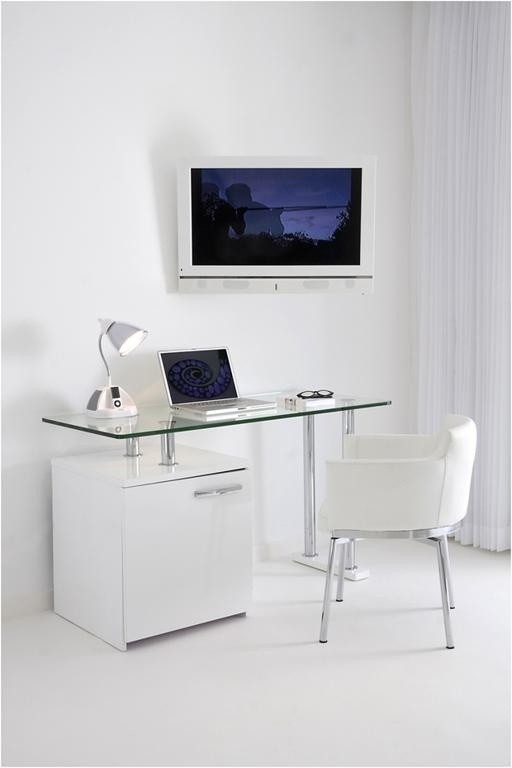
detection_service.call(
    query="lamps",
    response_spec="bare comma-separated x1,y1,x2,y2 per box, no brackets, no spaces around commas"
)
81,315,154,420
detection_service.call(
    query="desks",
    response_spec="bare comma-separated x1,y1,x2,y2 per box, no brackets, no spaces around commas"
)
38,381,394,658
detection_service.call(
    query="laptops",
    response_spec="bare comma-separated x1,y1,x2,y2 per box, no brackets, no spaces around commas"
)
157,346,277,415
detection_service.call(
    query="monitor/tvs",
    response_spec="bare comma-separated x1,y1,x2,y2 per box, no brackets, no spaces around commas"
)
178,155,375,278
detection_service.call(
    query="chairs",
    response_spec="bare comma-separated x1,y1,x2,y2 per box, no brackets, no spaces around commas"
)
313,411,482,653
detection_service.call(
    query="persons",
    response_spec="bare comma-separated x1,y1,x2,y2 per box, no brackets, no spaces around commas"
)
200,180,248,256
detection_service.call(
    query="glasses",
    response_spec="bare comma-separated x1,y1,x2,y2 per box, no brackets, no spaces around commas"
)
296,389,336,399
